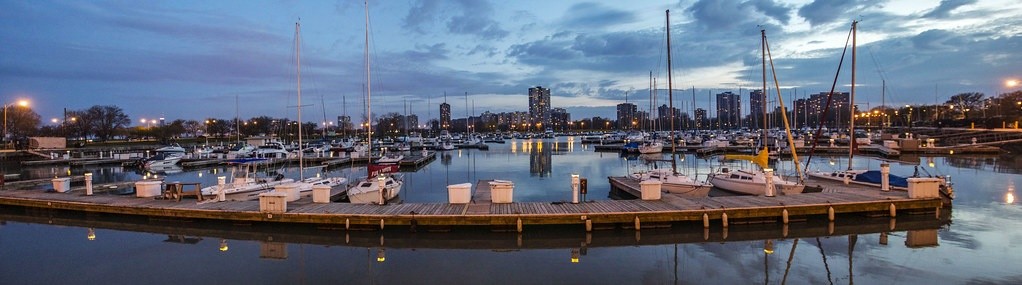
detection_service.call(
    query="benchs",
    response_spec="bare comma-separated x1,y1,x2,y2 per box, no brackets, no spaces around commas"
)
162,233,204,247
163,181,203,203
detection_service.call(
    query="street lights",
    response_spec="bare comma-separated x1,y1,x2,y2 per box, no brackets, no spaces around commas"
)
141,118,156,140
4,101,27,149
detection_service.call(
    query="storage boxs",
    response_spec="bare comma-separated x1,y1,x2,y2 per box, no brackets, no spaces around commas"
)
258,193,288,213
311,185,331,203
639,181,663,201
135,181,162,198
258,242,289,262
906,178,942,198
488,180,515,204
51,178,71,193
447,183,472,204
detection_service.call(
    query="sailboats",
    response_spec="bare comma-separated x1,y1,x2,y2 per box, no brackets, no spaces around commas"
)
803,19,955,208
707,28,807,197
627,7,714,197
345,1,403,205
268,18,349,204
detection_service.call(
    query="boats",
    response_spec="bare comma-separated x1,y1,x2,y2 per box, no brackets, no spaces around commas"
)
143,89,484,174
199,94,295,202
581,69,939,154
